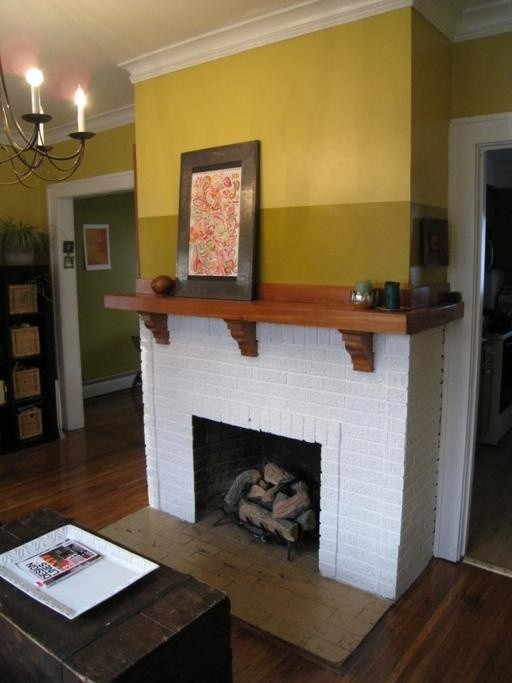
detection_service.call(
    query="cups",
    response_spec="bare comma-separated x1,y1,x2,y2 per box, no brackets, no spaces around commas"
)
384,281,400,309
374,288,384,308
356,280,371,293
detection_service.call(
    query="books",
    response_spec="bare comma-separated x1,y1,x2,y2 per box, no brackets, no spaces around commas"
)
16,538,103,589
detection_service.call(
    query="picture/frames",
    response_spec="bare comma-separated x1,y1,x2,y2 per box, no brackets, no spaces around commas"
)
174,139,260,301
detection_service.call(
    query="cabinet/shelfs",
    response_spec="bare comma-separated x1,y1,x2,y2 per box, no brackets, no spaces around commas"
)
0,265,58,456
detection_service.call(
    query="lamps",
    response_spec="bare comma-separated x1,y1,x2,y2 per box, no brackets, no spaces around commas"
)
0,55,95,187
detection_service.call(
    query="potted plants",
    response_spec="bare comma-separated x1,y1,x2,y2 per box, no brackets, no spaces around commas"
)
0,218,42,264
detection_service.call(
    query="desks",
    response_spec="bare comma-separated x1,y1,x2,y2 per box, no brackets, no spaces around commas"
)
0,507,231,681
